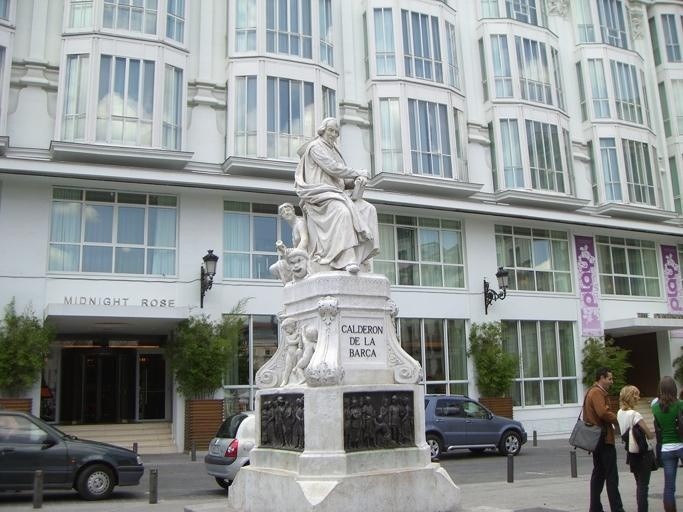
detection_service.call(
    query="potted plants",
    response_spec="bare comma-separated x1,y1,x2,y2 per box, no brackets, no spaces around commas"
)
579,335,632,412
161,295,255,452
466,321,520,421
0,294,52,414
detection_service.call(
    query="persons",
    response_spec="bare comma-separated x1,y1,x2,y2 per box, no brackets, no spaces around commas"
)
581,365,627,512
260,393,413,449
646,375,683,512
650,396,664,467
268,202,306,285
277,318,301,386
615,383,656,512
290,323,318,384
297,117,376,272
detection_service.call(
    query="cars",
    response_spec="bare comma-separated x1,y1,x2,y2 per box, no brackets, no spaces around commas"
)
424,393,528,462
204,408,258,494
0,408,145,501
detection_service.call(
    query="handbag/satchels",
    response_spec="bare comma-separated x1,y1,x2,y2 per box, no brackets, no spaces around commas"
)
628,410,640,454
646,440,659,472
569,419,602,452
675,409,683,441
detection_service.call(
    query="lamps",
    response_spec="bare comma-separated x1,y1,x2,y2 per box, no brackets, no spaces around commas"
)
199,248,218,310
481,266,509,314
